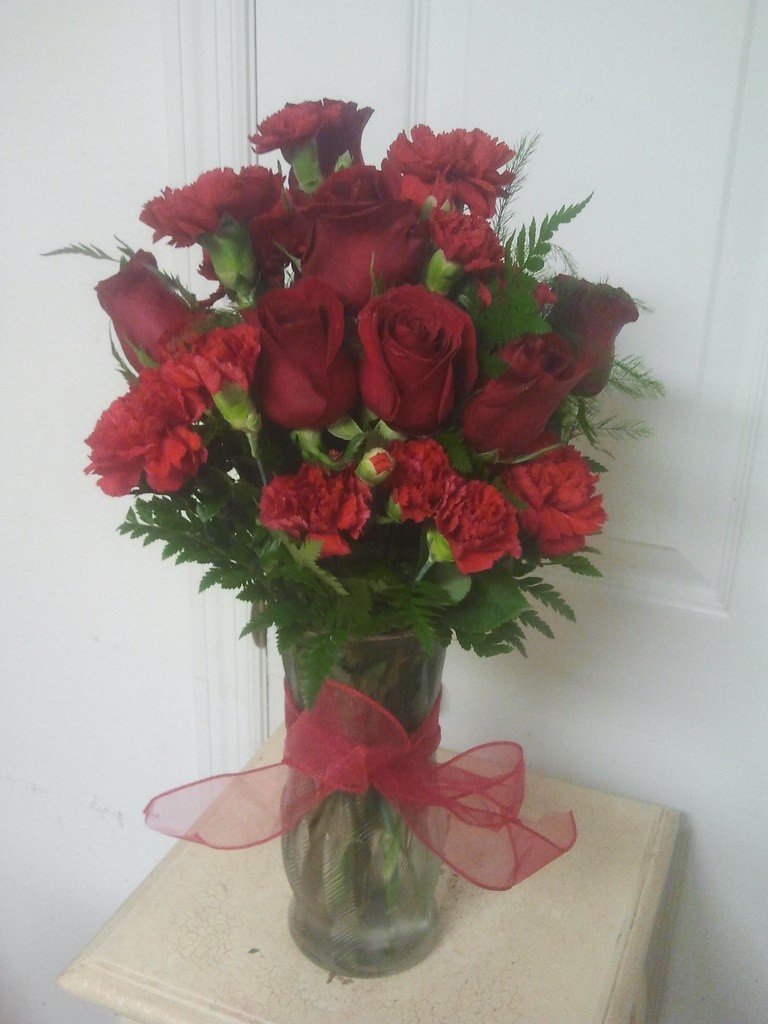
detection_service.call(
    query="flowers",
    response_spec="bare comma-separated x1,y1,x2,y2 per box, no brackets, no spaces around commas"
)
55,106,676,920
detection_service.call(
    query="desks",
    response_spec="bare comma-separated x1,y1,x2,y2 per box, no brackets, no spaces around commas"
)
57,744,687,1024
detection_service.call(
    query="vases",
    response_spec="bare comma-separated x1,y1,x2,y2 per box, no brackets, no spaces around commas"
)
274,620,443,977
